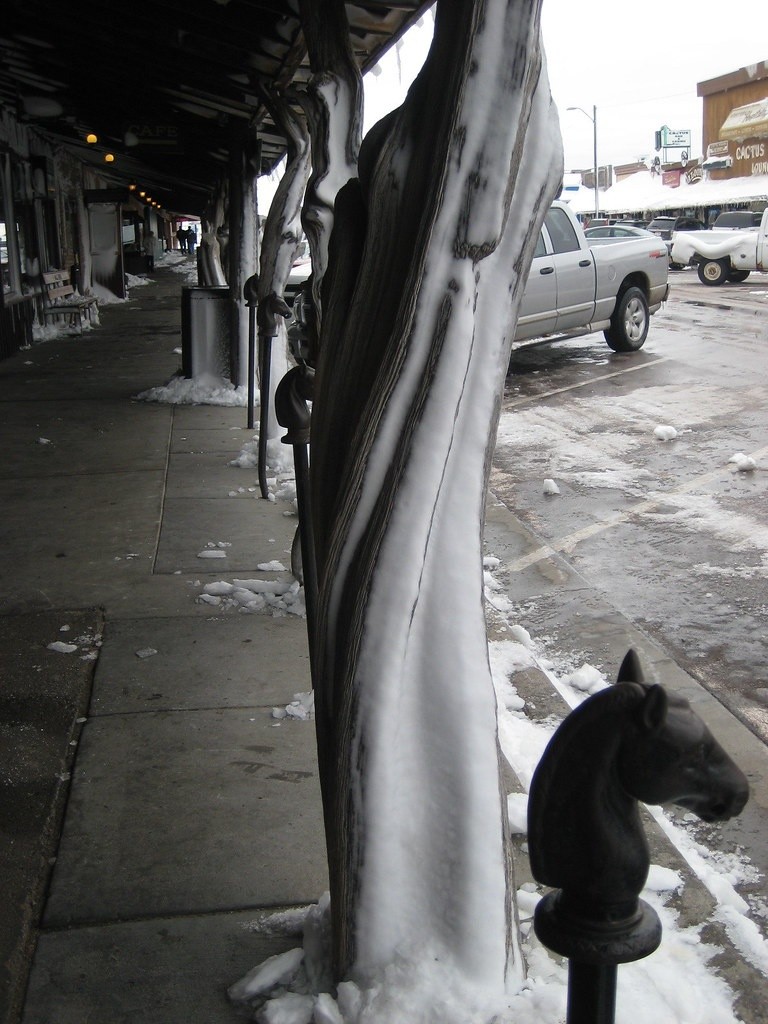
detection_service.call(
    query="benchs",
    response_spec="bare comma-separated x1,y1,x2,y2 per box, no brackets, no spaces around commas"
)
40,269,100,332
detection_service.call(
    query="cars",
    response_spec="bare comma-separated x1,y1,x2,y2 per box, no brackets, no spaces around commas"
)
284,232,312,307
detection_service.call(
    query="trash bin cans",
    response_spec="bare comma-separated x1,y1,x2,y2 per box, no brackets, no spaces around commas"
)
179,284,234,383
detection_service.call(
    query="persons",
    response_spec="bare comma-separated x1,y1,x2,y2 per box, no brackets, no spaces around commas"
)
176,226,187,255
185,226,195,254
142,231,155,268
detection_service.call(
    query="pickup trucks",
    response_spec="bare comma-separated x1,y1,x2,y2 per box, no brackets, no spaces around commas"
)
669,206,768,287
287,199,670,366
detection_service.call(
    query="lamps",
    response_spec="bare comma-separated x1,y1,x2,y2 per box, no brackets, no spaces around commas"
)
146,192,152,202
101,154,115,165
85,133,99,148
139,186,147,197
126,177,138,190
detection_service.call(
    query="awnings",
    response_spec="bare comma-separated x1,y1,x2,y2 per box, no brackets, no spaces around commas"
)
718,98,768,139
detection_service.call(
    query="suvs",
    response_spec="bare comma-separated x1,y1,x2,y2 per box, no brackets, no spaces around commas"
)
567,216,709,270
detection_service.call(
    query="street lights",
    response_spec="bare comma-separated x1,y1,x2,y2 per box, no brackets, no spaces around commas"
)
566,105,599,219
